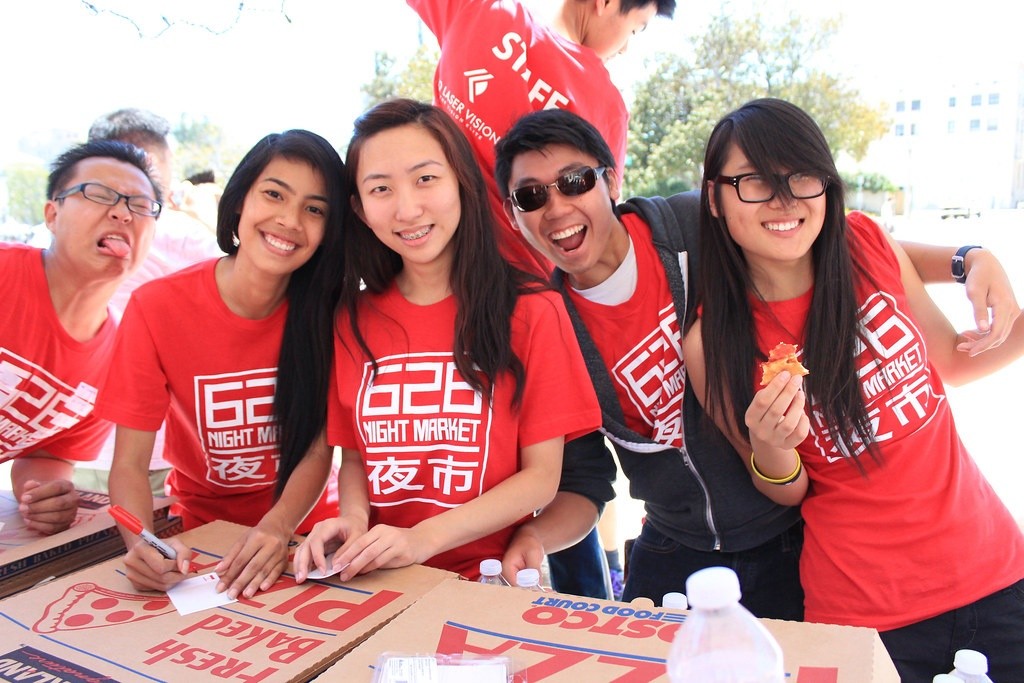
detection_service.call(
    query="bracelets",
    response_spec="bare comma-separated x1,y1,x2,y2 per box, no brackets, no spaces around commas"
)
751,451,802,485
951,246,982,283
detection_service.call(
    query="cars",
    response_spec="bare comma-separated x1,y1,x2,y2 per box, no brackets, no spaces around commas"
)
940,199,982,220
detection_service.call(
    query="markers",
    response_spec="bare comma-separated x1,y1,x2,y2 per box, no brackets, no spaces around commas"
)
107,505,197,575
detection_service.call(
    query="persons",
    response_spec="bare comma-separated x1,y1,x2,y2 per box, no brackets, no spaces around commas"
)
677,99,1024,683
409,0,675,599
25,105,228,501
91,128,349,598
495,111,1023,620
0,137,164,535
291,98,604,582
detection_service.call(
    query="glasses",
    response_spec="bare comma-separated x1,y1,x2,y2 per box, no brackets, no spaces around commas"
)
712,168,831,203
52,182,163,220
507,165,607,212
